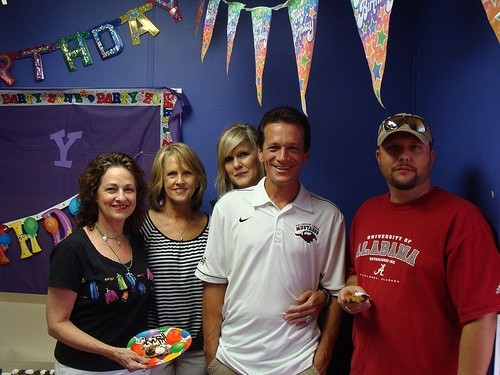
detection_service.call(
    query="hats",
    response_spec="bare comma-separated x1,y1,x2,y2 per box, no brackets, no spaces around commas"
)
377,112,432,146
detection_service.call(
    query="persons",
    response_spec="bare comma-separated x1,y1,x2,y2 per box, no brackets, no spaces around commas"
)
195,106,345,375
46,153,155,375
135,143,211,375
337,113,500,375
210,121,332,326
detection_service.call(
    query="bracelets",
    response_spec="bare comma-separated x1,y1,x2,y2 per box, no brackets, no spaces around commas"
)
319,289,330,307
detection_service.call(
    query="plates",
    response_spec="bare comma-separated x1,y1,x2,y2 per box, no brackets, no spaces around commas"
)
126,326,192,368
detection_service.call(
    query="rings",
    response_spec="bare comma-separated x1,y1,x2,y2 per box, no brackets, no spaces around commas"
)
307,315,312,324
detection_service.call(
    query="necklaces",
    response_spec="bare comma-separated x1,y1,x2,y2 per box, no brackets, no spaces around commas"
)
94,223,133,276
164,211,190,241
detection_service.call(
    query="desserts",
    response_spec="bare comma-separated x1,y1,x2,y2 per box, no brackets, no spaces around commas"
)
142,333,168,358
344,291,372,308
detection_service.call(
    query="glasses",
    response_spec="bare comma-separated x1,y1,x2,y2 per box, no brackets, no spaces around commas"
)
378,115,428,133
